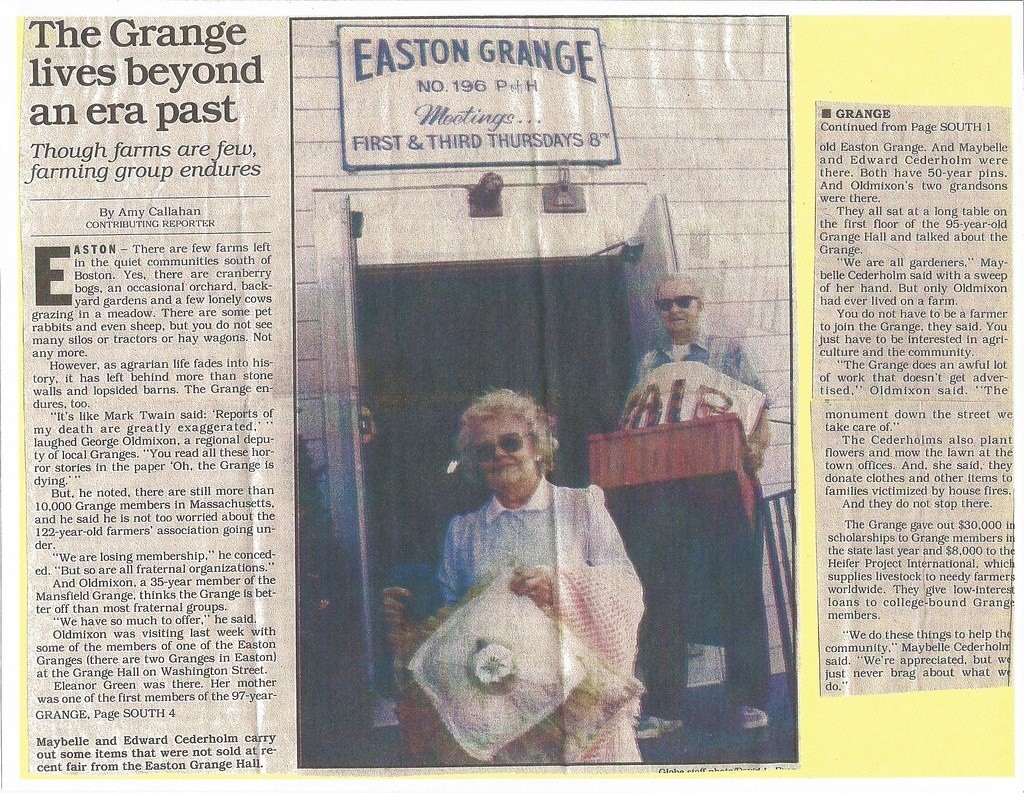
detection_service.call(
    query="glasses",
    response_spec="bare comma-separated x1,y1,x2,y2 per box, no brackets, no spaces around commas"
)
471,431,530,465
653,294,699,312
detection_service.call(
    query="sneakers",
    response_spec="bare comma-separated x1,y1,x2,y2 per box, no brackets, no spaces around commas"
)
635,715,685,740
740,705,769,730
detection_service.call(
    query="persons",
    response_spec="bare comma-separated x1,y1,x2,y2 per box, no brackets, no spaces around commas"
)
635,273,770,741
381,388,646,764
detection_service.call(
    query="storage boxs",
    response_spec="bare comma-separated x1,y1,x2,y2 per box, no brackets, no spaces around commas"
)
587,412,766,549
